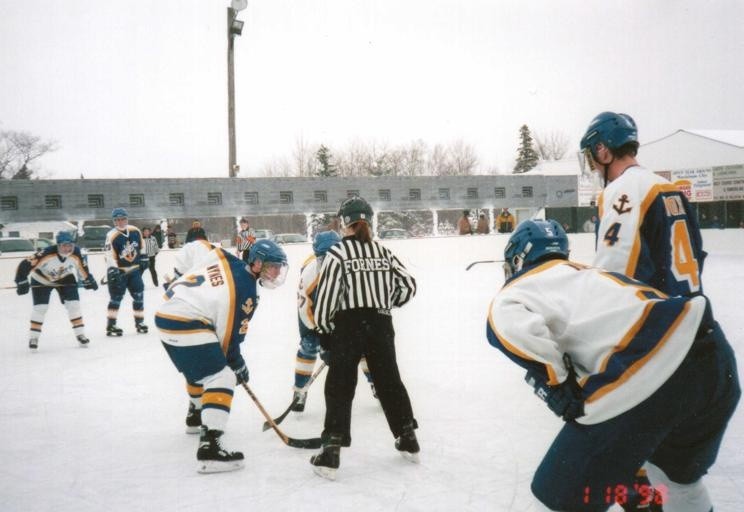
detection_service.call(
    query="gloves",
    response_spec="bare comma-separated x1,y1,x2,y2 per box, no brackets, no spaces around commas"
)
140,257,152,273
15,279,29,295
84,275,98,291
235,365,248,387
106,266,119,281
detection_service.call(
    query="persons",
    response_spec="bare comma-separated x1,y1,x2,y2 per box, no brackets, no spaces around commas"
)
309,196,422,481
495,208,516,233
477,214,489,234
168,226,176,248
235,218,257,261
291,228,384,412
583,215,597,233
103,207,149,336
561,221,573,233
14,228,98,353
139,226,160,288
151,225,163,249
154,238,289,474
458,210,473,234
185,219,207,243
575,110,710,298
486,218,743,511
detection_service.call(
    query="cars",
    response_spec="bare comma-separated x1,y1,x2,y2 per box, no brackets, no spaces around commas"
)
255,229,274,243
27,237,54,249
380,228,409,238
80,225,112,253
274,233,308,244
0,237,38,261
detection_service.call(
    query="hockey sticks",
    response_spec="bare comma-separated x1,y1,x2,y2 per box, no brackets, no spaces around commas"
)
101,267,144,283
262,363,327,431
240,378,321,449
466,258,504,271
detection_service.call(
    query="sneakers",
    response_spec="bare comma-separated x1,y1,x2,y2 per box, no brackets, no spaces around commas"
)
106,320,122,332
197,430,244,462
291,390,306,412
29,338,38,349
394,431,419,454
186,410,203,429
76,333,90,344
309,444,341,470
135,321,148,330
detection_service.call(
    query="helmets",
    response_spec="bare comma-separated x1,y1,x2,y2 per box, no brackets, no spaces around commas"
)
502,220,570,278
337,197,374,230
246,240,288,289
313,231,341,256
56,231,76,256
113,209,127,229
575,111,639,178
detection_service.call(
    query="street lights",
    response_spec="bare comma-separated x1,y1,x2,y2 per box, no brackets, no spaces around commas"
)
227,0,246,178
